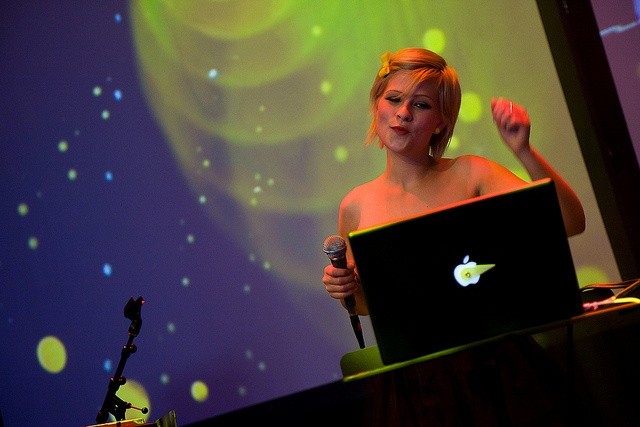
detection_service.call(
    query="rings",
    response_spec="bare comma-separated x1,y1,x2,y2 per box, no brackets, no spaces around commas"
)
509,101,513,112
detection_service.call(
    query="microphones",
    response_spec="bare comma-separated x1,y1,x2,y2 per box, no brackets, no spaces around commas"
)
323,234,365,349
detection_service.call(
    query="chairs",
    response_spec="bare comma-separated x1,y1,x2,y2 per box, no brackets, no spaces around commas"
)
340,344,385,377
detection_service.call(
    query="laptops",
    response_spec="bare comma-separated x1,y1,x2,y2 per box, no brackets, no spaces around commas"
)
348,176,586,366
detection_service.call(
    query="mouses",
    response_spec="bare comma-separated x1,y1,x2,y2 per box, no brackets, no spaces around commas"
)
582,288,615,310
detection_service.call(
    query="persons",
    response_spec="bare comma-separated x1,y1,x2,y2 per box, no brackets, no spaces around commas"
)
322,47,586,317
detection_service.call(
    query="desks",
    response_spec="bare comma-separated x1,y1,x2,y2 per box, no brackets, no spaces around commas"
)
178,295,640,427
